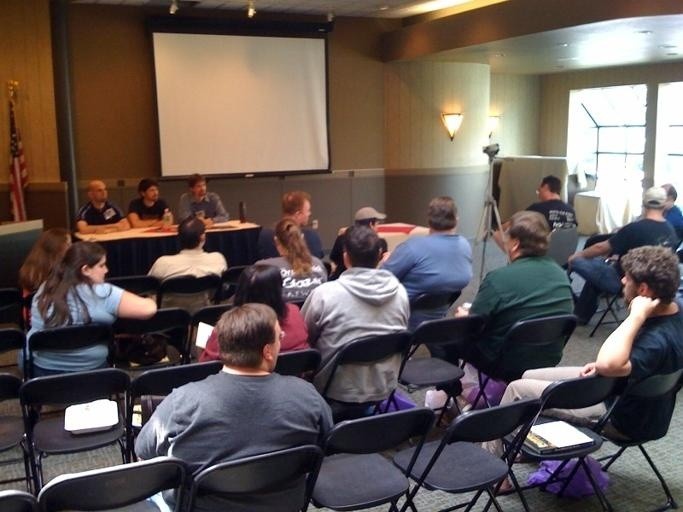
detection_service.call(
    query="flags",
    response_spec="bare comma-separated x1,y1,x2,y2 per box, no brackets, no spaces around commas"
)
8,98,29,222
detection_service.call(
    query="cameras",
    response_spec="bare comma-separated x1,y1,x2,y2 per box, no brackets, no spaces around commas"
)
484,142,499,155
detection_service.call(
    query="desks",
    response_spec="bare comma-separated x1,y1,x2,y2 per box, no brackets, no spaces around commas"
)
74,220,261,277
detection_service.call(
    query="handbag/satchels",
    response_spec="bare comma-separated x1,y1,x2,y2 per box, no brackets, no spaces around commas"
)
112,329,169,365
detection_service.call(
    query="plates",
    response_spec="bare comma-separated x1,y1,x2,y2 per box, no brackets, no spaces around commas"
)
64,399,119,435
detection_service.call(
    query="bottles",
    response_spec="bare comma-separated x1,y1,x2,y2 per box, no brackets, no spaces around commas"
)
162,208,173,229
239,201,247,223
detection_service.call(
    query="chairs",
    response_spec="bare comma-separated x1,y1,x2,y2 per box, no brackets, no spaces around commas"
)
1,264,322,512
409,289,462,312
311,314,681,511
547,223,681,338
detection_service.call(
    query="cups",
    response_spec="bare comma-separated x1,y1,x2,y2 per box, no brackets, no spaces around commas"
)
462,303,472,311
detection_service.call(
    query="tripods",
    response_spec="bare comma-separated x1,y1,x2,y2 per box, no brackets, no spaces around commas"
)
468,159,513,285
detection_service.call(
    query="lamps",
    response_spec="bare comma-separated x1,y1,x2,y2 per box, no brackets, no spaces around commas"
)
441,112,463,141
487,116,500,138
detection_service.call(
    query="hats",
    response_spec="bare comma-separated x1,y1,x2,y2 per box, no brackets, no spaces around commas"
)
644,187,670,208
354,206,388,221
